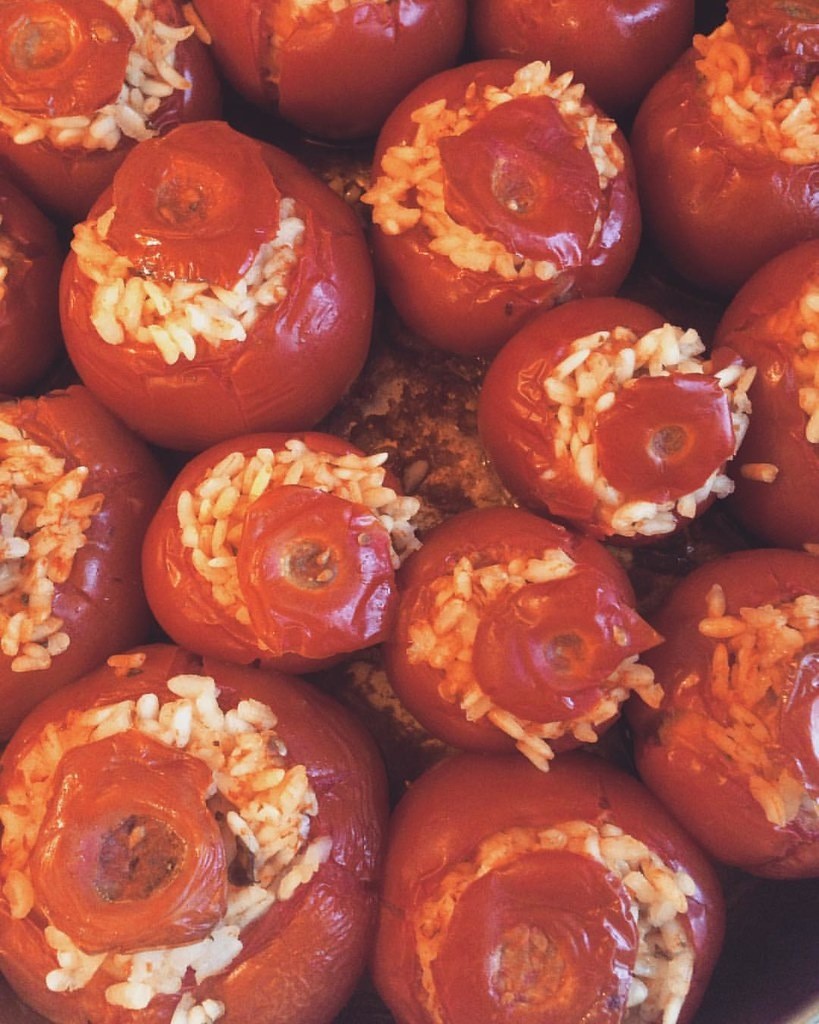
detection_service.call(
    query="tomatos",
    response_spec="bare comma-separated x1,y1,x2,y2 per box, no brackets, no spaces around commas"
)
0,0,819,1024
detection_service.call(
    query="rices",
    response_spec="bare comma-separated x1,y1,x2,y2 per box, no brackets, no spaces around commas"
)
1,0,819,1024
